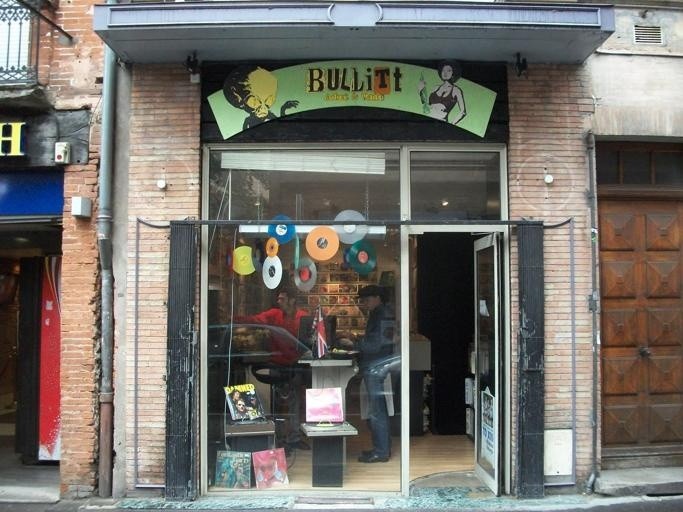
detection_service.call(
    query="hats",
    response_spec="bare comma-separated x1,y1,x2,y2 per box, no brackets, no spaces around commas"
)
342,344,400,435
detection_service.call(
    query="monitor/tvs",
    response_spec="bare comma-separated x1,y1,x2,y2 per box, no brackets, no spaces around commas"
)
214,449,251,490
304,386,343,424
252,447,289,490
298,422,358,436
221,382,265,421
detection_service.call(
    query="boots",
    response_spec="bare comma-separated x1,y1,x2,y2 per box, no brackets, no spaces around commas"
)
206,321,315,412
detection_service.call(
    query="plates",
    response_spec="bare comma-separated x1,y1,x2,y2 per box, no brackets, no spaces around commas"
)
300,422,358,488
226,419,275,487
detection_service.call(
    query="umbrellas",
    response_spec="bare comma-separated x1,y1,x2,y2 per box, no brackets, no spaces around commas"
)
283,439,311,450
358,452,388,463
362,448,391,457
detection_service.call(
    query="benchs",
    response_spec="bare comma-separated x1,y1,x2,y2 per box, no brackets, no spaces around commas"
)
311,303,327,358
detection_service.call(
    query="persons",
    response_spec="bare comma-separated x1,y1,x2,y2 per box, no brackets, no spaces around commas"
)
353,284,401,465
228,388,260,420
228,289,318,452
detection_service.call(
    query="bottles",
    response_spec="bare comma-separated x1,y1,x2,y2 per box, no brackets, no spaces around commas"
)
356,285,382,299
